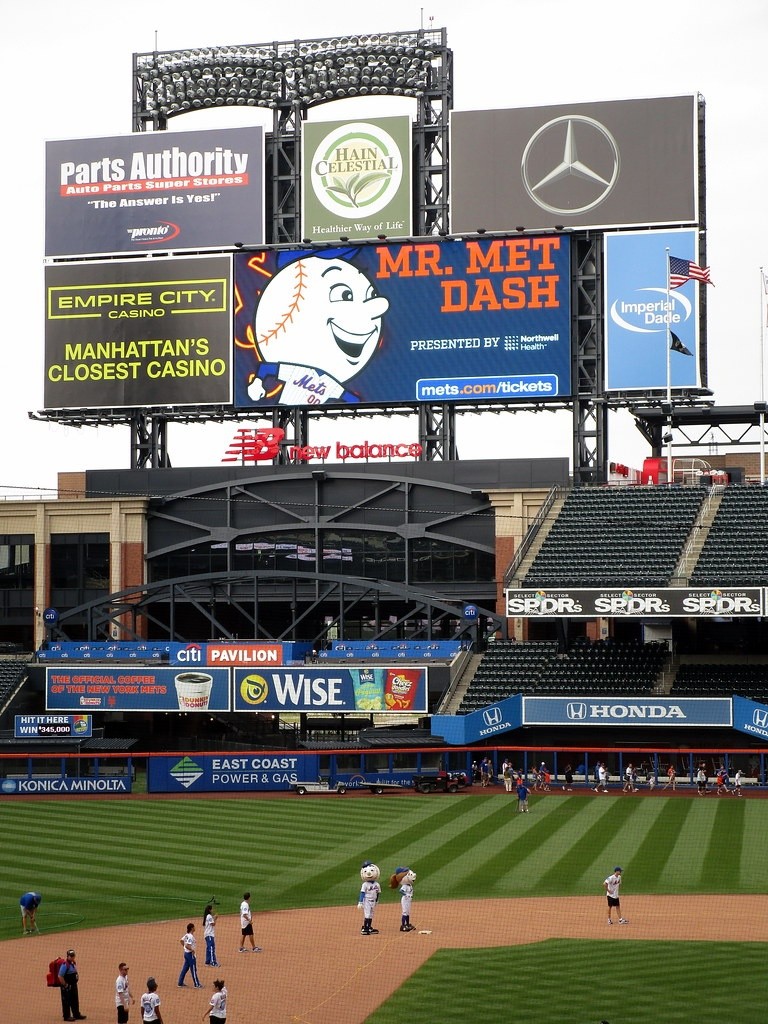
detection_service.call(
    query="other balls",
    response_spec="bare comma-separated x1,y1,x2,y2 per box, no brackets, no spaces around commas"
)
30,930,32,933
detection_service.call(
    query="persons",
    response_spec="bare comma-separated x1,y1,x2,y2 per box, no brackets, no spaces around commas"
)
715,764,731,796
237,893,263,953
591,760,610,792
176,922,203,988
622,763,638,793
202,905,221,967
731,770,745,797
356,861,381,934
661,764,677,791
389,866,417,931
649,775,655,791
601,867,629,924
696,761,712,797
114,962,136,1024
202,978,228,1024
471,757,551,793
561,765,573,791
58,949,86,1022
19,892,41,935
516,783,532,813
140,976,164,1024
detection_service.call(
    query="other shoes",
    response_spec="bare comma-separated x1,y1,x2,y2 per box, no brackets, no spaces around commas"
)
73,1013,86,1020
471,780,744,813
30,930,34,933
360,926,369,935
406,924,416,930
608,920,612,925
619,919,629,924
23,931,29,935
64,1016,76,1021
368,927,379,934
400,925,410,932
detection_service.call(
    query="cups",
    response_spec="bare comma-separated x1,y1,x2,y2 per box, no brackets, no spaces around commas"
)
174,672,213,710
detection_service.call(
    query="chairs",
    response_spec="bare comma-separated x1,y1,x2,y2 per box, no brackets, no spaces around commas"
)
456,483,768,716
0,659,30,704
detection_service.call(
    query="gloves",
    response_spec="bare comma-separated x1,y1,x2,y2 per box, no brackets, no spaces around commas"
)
358,902,363,909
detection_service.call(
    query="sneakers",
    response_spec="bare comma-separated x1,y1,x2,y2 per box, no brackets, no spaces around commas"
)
204,962,212,967
177,983,188,988
252,947,262,953
239,947,249,952
212,964,221,968
194,984,205,989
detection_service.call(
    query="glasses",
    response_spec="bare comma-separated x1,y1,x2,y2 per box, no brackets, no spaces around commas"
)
123,968,129,971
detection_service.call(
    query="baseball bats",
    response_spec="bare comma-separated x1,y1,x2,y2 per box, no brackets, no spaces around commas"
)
34,921,43,935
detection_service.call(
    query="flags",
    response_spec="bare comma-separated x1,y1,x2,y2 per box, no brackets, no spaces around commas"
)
669,256,715,289
669,331,694,356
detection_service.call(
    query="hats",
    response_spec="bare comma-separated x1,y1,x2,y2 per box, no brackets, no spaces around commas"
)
541,762,545,765
615,867,623,872
35,895,41,904
67,949,75,957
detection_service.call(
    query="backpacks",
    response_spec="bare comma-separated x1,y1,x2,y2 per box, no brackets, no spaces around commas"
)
46,957,70,987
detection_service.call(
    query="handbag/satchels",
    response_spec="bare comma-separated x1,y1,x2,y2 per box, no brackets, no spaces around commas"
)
624,775,630,781
